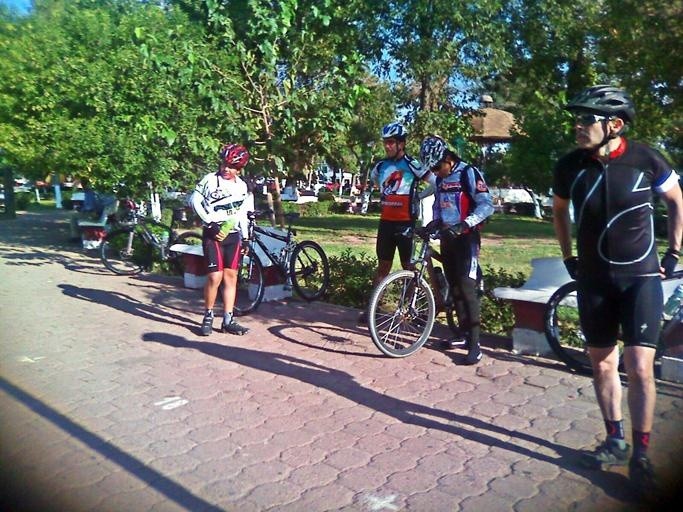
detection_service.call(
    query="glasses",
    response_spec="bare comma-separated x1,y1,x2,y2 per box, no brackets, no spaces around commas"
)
430,155,446,171
382,140,396,145
222,161,236,169
572,110,618,125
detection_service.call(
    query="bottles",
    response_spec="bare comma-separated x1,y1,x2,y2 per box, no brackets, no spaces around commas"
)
663,283,683,317
434,266,447,301
216,217,235,241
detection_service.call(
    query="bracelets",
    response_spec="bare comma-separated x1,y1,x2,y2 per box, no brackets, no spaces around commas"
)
667,248,683,257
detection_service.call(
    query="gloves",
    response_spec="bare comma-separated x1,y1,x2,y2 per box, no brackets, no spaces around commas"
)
445,223,464,240
426,220,440,231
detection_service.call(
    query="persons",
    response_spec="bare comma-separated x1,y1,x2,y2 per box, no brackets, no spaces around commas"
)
71,177,97,239
189,144,250,335
552,84,683,501
360,123,438,323
420,134,495,365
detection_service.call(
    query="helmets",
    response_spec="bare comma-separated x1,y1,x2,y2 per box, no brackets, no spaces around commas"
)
566,84,635,123
382,122,407,139
221,144,248,168
420,135,446,167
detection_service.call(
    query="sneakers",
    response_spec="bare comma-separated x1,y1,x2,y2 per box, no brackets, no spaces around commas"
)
629,457,653,494
221,323,250,335
578,441,629,465
465,351,482,364
201,318,212,335
360,310,380,322
449,336,480,348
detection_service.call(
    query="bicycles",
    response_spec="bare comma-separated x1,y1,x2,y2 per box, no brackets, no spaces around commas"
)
99,198,203,278
218,209,329,316
543,251,682,376
366,225,484,359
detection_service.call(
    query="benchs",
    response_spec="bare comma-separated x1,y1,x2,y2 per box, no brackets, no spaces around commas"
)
169,226,292,303
76,208,110,249
494,257,683,386
70,193,85,209
